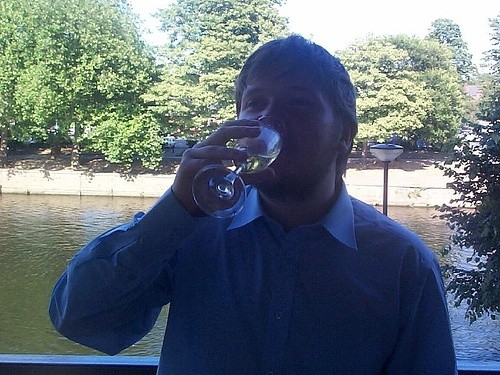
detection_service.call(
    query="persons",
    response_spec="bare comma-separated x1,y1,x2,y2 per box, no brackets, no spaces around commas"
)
51,36,458,375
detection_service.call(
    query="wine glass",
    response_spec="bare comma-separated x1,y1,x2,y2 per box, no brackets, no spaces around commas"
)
192,122,282,218
